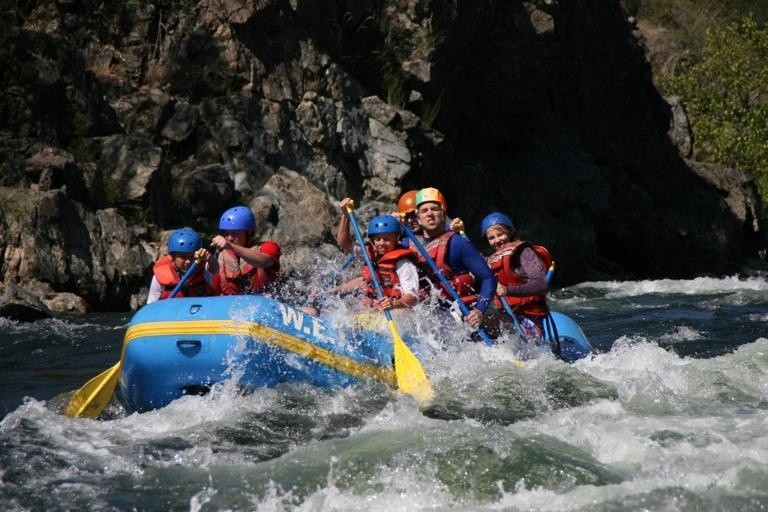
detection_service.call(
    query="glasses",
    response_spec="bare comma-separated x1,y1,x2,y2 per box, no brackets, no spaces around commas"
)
404,213,416,219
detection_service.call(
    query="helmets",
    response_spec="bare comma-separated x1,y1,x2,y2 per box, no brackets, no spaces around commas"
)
415,187,447,211
398,190,418,216
368,215,402,238
168,228,202,254
481,212,513,233
219,206,256,241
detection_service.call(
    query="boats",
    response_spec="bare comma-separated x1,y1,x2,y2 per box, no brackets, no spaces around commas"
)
117,293,593,415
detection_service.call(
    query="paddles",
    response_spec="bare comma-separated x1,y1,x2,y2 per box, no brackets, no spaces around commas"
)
339,200,431,401
64,254,204,419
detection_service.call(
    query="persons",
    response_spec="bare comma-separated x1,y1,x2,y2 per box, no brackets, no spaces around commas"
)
449,212,547,344
398,187,496,328
337,197,419,311
209,206,281,296
146,227,219,305
396,190,421,233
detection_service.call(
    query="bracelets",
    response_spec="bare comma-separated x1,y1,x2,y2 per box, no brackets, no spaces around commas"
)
353,245,358,257
392,297,395,308
